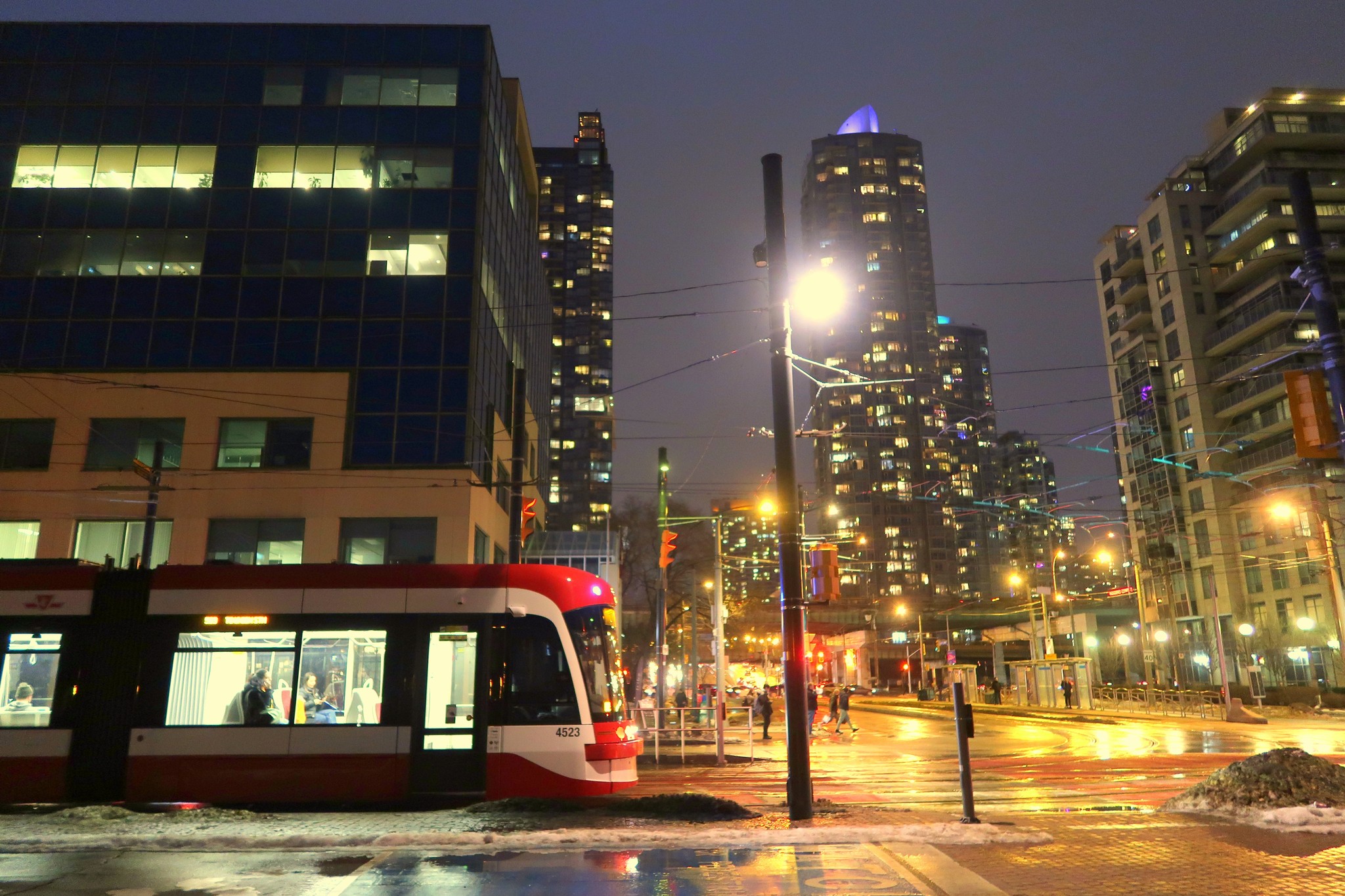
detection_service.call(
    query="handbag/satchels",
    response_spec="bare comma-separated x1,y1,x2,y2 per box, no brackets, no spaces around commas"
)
823,715,832,724
272,679,292,720
754,694,764,714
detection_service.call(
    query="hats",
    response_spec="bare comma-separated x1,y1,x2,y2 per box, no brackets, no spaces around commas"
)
15,683,33,698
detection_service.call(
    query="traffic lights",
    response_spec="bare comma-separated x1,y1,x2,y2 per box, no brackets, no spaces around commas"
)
846,650,855,669
818,651,824,663
924,664,930,671
520,495,536,549
816,664,823,670
903,663,908,670
1221,686,1225,697
659,529,678,568
1256,655,1266,664
806,651,812,663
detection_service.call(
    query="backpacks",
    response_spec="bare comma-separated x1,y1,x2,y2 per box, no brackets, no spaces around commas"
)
742,698,753,711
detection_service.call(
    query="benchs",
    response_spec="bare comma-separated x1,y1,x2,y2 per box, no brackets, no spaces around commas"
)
0,707,51,727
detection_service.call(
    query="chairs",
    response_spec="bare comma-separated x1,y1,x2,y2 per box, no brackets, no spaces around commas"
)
344,678,382,724
272,679,293,724
222,693,245,724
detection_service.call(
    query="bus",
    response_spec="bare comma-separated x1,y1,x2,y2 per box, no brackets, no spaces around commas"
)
0,557,645,801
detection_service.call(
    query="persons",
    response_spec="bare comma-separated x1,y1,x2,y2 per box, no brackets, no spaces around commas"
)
1061,677,1072,709
818,689,840,732
298,671,337,723
652,686,657,699
757,684,773,739
241,669,282,725
675,688,688,719
835,688,859,735
745,689,756,735
903,684,909,695
4,682,37,711
803,682,818,734
991,677,1001,705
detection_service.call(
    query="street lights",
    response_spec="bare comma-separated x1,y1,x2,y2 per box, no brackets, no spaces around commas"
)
743,634,781,666
1085,637,1102,685
1296,617,1318,689
896,604,912,693
1097,532,1233,718
657,446,673,741
753,152,848,819
715,496,783,767
1052,550,1079,659
1154,631,1167,690
1117,634,1132,689
1238,623,1253,667
1107,532,1132,601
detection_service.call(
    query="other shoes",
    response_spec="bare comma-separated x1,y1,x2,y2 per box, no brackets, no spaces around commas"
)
853,728,859,733
835,730,843,734
809,734,816,738
763,735,772,739
1070,707,1073,709
